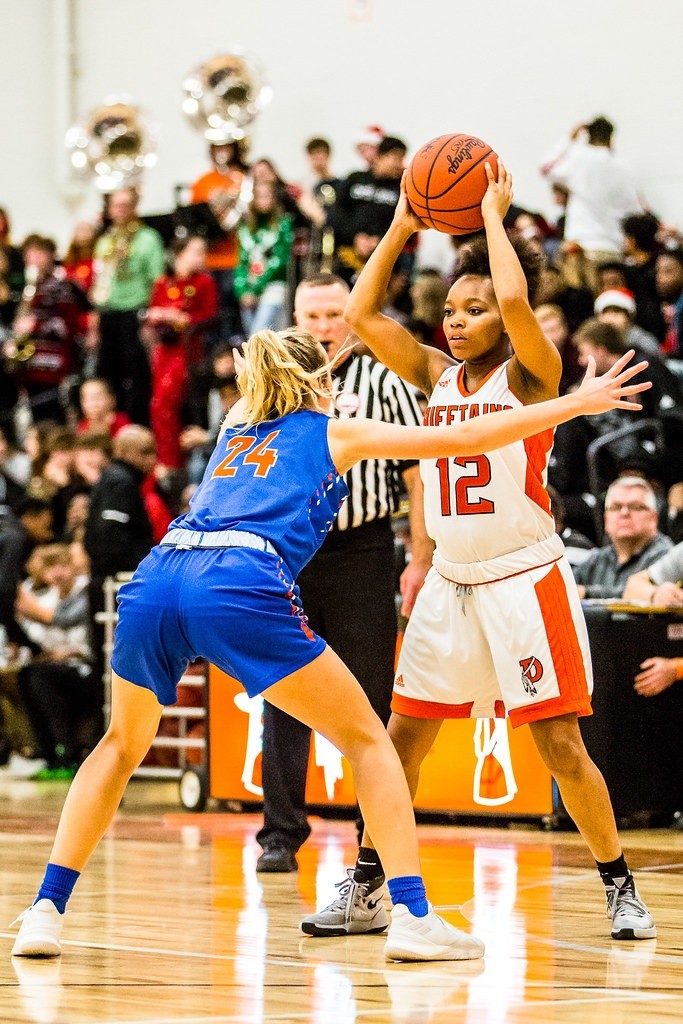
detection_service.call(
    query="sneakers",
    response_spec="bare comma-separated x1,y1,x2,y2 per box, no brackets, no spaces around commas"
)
384,901,485,960
10,899,66,956
301,869,393,937
600,869,657,939
256,838,298,872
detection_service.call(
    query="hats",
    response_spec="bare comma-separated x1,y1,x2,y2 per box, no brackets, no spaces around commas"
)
357,127,385,147
624,215,659,252
593,286,636,314
567,243,584,256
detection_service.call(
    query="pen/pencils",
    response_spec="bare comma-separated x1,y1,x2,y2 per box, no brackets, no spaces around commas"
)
675,580,683,588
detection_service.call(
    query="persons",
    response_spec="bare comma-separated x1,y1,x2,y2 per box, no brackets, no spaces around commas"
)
1,115,683,871
293,163,653,940
14,328,656,960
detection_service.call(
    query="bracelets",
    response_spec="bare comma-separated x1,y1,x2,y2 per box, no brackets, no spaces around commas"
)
649,591,656,604
674,656,683,680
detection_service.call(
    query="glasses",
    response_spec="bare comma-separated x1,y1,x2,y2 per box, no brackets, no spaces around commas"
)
604,501,650,515
140,446,156,455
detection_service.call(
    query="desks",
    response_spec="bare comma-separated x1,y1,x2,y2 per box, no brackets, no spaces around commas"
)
557,598,683,831
205,602,565,830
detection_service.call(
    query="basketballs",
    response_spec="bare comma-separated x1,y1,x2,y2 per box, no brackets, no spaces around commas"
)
406,132,500,236
